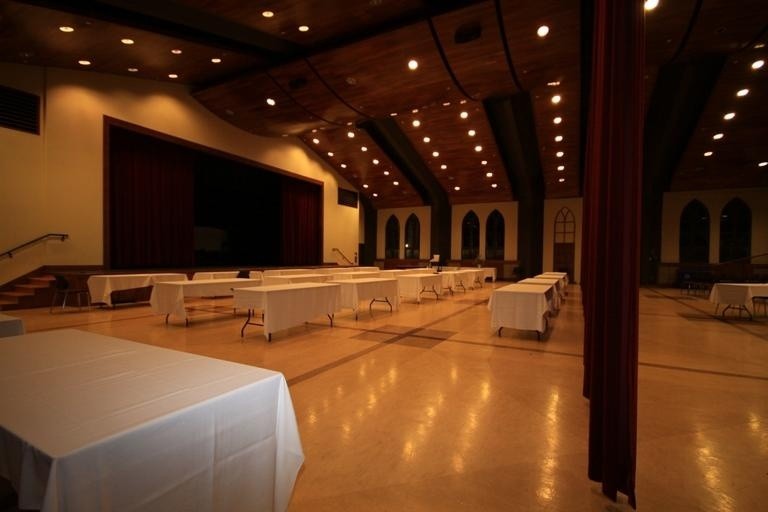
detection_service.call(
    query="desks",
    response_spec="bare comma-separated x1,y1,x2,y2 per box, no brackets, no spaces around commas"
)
493,271,569,341
0,329,307,512
0,313,23,337
710,282,768,323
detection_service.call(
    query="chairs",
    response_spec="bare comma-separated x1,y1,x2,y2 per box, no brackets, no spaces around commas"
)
429,254,441,268
50,275,91,313
